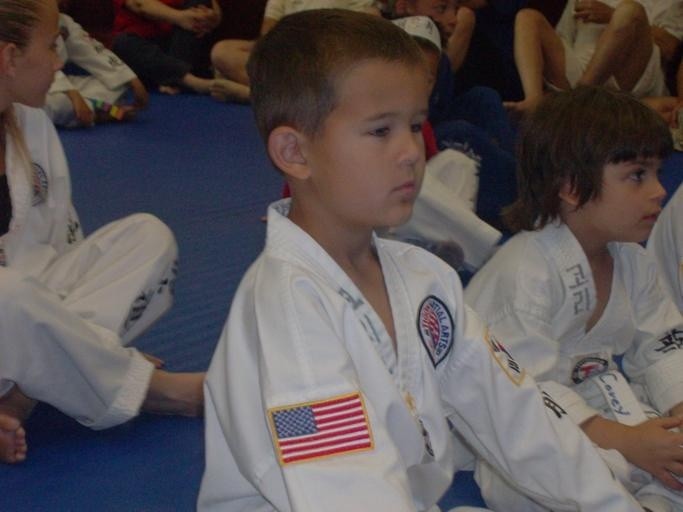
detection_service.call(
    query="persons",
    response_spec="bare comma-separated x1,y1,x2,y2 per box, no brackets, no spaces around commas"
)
464,86,683,512
45,1,683,317
197,8,647,512
1,1,207,463
